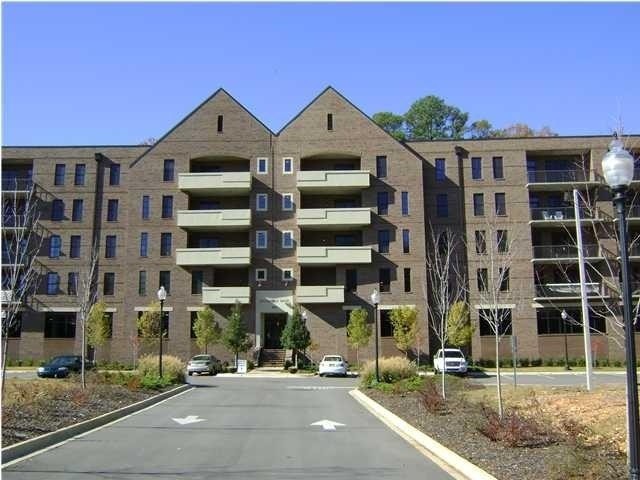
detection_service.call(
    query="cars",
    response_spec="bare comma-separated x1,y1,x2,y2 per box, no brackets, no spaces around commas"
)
186,354,222,375
318,354,348,378
433,348,468,374
36,355,94,378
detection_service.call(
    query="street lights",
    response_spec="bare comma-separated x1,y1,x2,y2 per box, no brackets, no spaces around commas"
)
156,286,167,376
371,288,380,377
601,131,640,470
